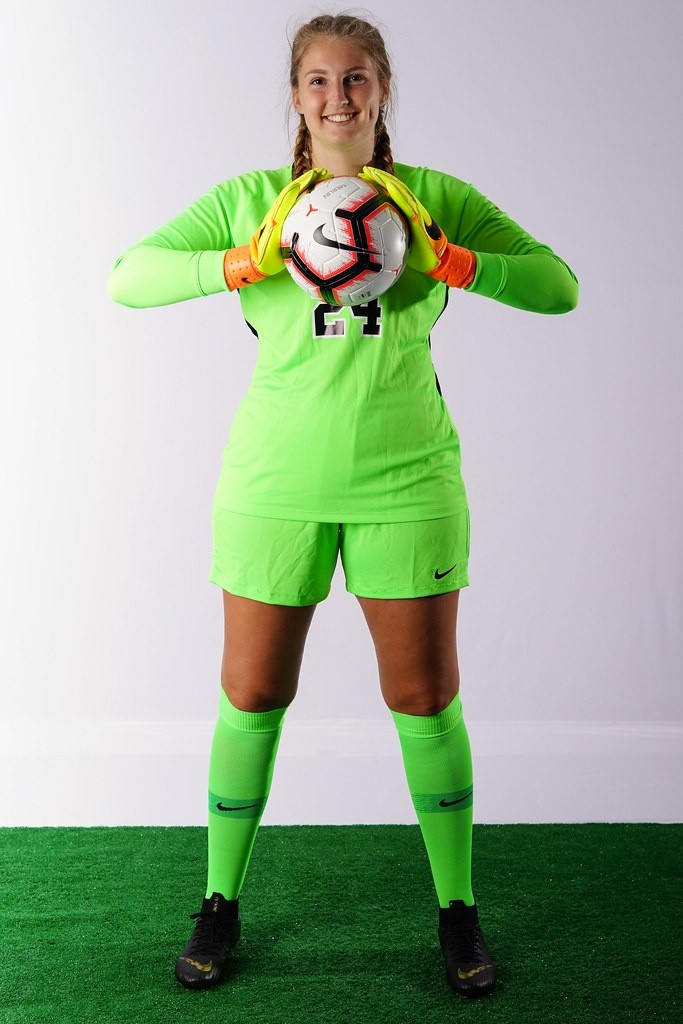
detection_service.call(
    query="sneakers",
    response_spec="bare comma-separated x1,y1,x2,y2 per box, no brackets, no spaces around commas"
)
175,894,241,987
437,901,497,998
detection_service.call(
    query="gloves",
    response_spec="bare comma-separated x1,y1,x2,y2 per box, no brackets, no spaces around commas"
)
249,165,334,277
357,166,446,271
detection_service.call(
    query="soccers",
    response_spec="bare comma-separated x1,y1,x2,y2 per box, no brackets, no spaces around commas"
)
280,179,404,305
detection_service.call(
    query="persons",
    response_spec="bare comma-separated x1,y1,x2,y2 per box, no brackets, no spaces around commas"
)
104,12,579,996
